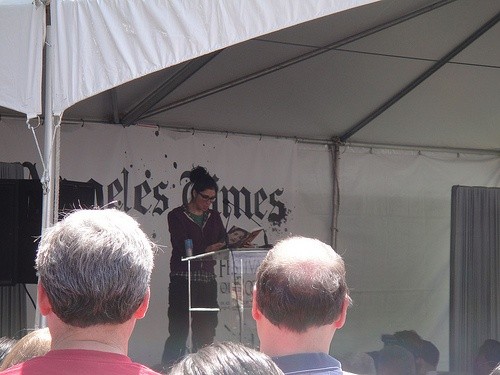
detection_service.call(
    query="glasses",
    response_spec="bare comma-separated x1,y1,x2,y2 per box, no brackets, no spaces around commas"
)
198,191,216,202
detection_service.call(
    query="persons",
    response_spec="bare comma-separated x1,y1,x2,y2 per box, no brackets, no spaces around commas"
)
0,207,164,375
249,233,355,375
226,229,245,247
162,165,256,375
0,328,500,375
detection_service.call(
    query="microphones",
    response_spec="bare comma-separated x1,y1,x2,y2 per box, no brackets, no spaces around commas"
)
219,198,273,250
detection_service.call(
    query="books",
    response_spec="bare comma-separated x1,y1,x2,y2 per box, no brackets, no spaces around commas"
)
222,225,264,247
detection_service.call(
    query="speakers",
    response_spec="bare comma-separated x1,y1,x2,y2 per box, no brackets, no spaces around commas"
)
0,181,43,285
59,179,96,222
